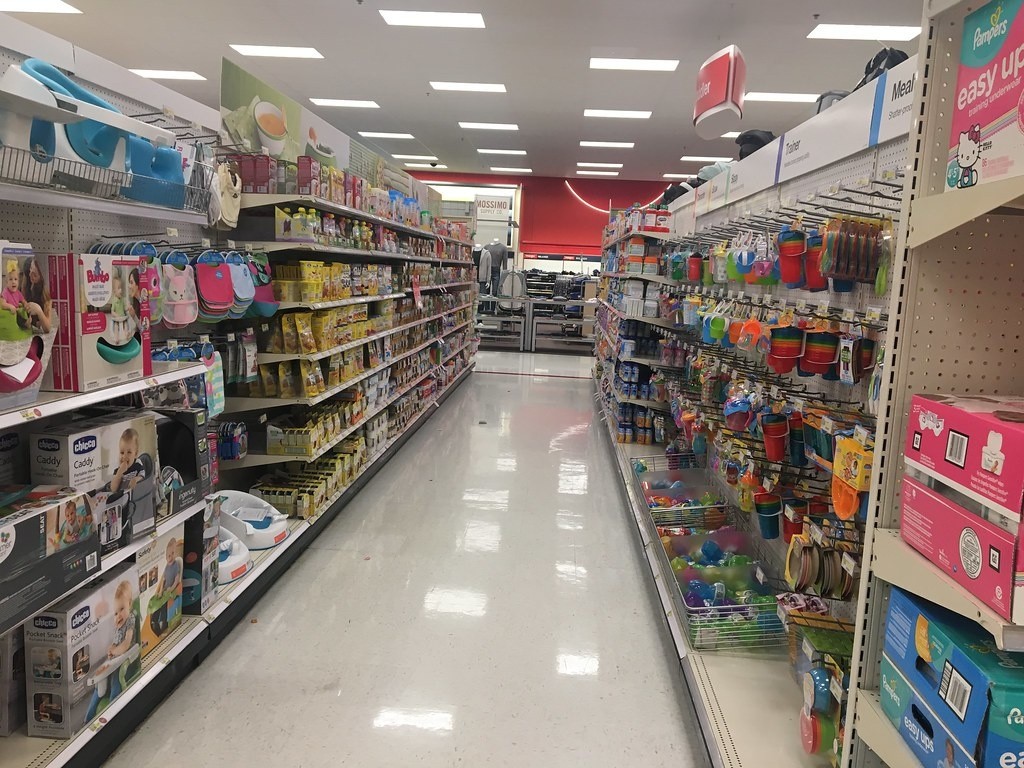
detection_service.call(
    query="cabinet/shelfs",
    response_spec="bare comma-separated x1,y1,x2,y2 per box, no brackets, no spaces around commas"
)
0,145,312,768
236,195,440,536
589,189,697,476
435,218,480,407
477,270,599,354
832,0,1024,766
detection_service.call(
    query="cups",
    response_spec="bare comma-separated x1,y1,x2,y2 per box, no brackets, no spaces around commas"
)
754,494,828,544
253,100,287,160
724,230,829,291
723,398,809,467
687,257,713,285
701,313,840,381
761,411,808,469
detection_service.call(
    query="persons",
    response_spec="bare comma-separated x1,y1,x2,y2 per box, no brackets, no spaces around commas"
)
37,649,61,724
111,428,145,492
103,509,117,543
472,238,508,311
141,570,157,592
158,537,181,600
205,501,220,554
111,268,141,346
0,256,52,333
64,502,92,542
82,581,135,726
591,269,600,276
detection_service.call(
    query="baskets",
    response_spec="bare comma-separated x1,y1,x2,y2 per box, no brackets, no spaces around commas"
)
629,453,729,527
647,505,796,650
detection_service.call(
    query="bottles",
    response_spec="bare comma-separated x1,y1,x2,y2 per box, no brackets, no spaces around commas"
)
284,206,399,254
629,202,672,233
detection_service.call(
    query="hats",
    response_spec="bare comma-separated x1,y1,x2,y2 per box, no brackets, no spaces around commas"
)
220,167,242,229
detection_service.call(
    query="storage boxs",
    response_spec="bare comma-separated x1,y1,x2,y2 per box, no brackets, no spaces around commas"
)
629,454,728,524
0,238,222,739
646,505,801,651
876,0,1023,768
